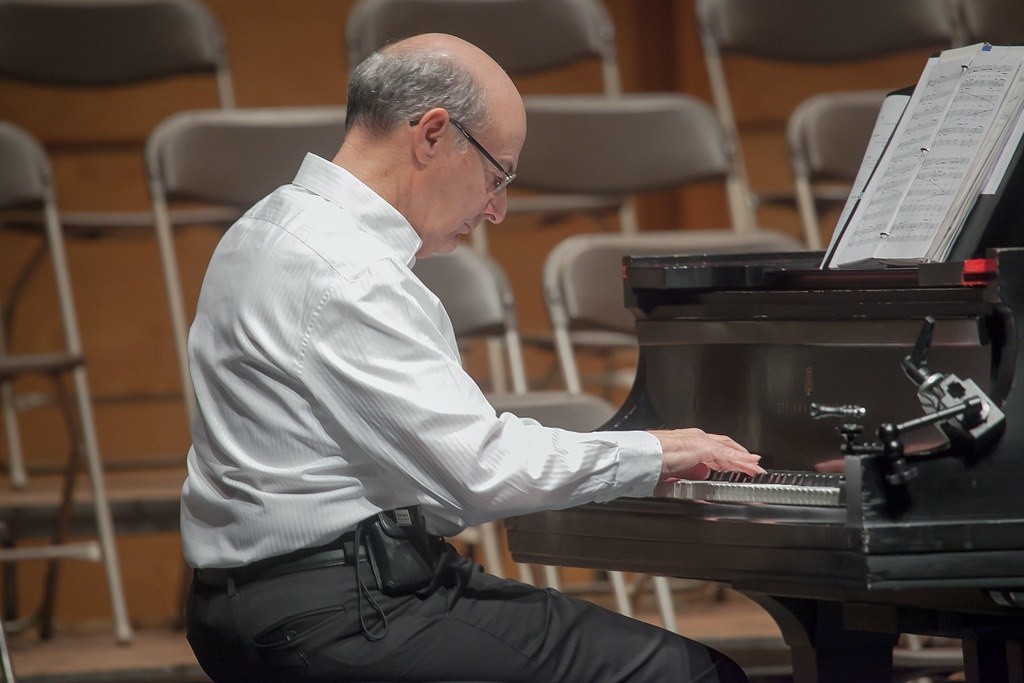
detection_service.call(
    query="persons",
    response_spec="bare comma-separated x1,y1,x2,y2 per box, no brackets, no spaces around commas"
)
180,33,769,683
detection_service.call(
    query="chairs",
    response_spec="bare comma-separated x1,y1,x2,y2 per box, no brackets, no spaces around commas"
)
0,0,1024,683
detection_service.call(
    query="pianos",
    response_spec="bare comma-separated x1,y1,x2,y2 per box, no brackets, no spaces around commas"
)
504,251,1021,683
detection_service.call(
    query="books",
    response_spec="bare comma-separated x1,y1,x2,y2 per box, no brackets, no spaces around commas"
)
825,40,1024,268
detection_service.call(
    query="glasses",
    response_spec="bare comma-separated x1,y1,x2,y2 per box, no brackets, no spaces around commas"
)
410,117,516,192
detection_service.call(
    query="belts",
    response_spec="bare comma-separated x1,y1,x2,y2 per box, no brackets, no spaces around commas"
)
192,541,371,586
